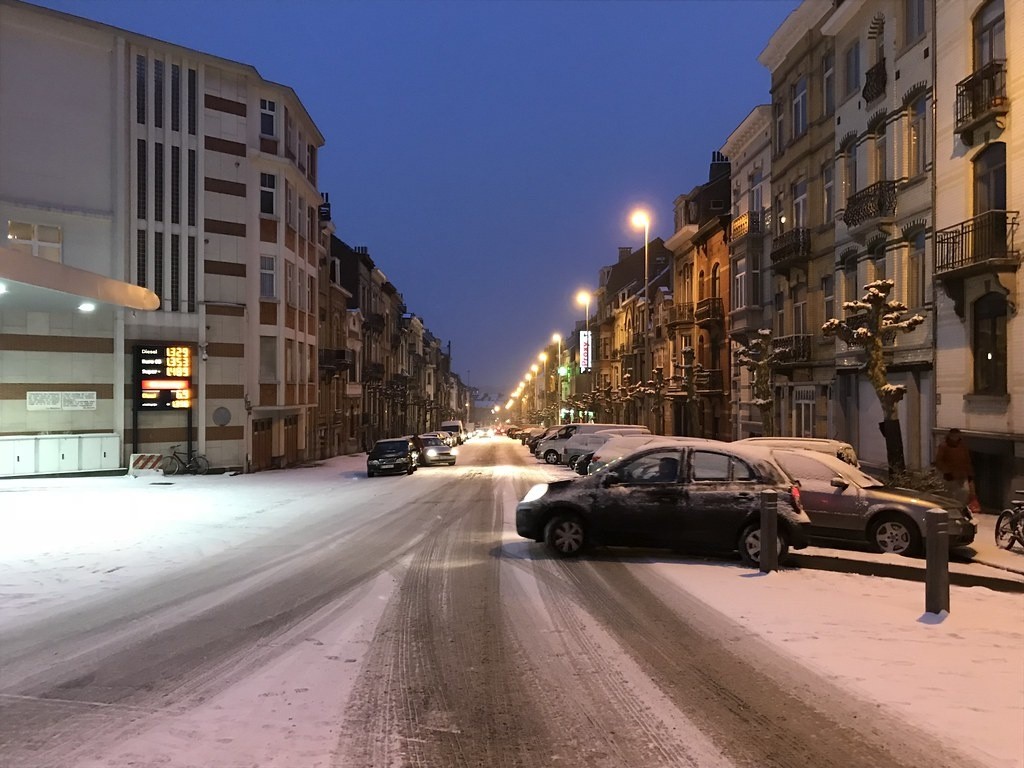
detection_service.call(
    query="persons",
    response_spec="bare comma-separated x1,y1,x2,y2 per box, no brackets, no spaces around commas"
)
410,433,424,451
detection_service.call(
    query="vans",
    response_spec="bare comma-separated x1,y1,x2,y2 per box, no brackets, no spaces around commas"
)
441,420,465,444
535,423,651,465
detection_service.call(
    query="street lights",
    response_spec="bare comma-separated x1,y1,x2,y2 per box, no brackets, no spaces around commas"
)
631,210,649,432
553,334,561,426
495,364,537,428
577,293,589,426
540,353,548,426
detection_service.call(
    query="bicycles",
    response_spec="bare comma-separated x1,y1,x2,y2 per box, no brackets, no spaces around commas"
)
159,444,209,476
995,490,1024,551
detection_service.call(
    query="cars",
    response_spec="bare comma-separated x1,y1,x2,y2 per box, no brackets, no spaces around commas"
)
516,442,815,572
562,430,730,477
507,425,564,454
494,428,503,435
479,427,493,439
366,438,417,478
414,431,457,466
464,426,477,439
732,437,978,558
